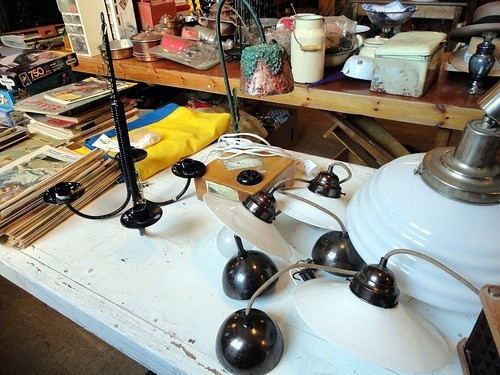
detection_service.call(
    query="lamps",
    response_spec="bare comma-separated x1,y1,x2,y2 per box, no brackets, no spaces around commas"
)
216,0,294,146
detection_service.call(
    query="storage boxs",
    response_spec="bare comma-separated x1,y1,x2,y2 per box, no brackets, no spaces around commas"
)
193,147,295,205
370,31,447,98
0,50,78,129
55,0,140,56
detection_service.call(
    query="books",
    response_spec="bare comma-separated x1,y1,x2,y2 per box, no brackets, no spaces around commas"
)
0,23,66,49
0,145,122,249
13,75,138,143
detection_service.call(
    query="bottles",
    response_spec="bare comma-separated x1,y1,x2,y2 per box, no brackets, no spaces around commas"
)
360,35,386,61
290,14,328,83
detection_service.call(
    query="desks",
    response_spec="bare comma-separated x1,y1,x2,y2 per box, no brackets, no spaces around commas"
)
0,0,500,375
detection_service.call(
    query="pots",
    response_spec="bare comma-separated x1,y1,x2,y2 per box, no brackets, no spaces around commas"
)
176,13,199,26
131,24,164,61
98,39,132,60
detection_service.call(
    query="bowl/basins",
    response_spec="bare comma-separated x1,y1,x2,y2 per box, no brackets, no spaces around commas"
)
363,6,419,29
325,34,365,68
237,18,279,43
